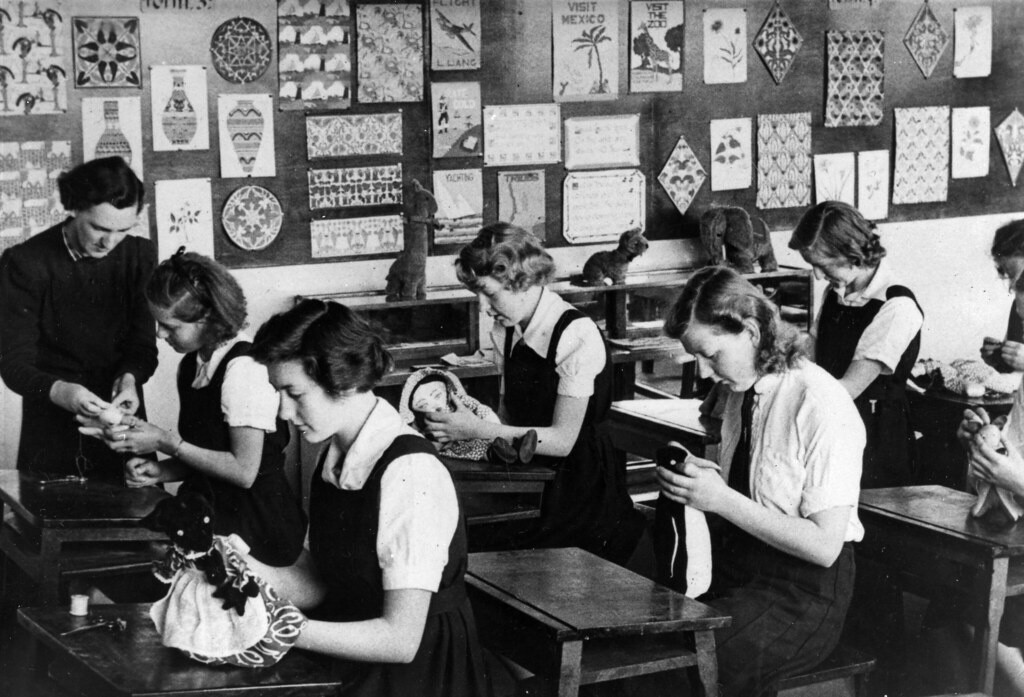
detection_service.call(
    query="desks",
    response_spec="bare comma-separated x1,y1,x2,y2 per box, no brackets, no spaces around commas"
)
0,400,1023,697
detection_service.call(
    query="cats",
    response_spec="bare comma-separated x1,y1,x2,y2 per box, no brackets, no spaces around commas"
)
572,228,649,283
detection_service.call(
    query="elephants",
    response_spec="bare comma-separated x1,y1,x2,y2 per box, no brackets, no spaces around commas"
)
697,207,778,274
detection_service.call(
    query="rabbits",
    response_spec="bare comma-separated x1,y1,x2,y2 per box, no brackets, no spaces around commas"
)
386,179,438,301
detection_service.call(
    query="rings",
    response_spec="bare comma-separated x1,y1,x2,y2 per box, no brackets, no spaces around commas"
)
123,433,126,441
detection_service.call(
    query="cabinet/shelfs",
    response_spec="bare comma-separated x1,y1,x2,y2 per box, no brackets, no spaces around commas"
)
294,261,816,400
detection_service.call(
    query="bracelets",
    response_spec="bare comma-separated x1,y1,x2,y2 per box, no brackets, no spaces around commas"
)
172,438,184,459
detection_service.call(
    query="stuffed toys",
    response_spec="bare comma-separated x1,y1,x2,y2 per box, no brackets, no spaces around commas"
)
384,177,438,300
569,229,649,286
701,206,782,272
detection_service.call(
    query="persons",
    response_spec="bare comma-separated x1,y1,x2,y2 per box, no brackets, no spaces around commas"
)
97,250,307,567
654,202,1024,697
208,299,498,697
399,365,537,465
426,223,643,564
0,158,160,485
